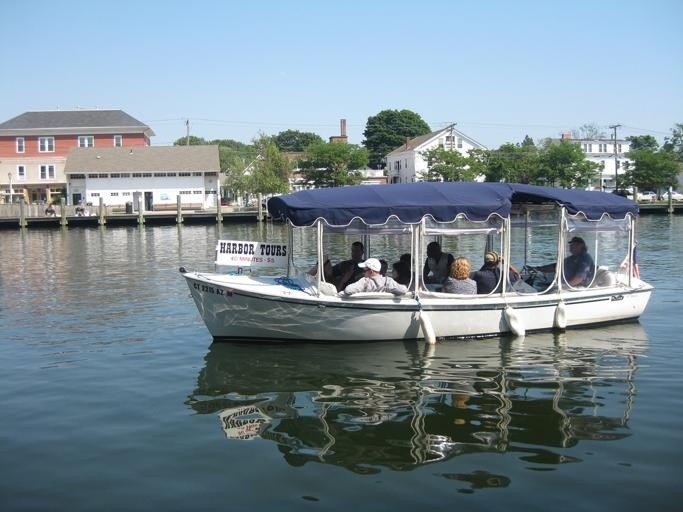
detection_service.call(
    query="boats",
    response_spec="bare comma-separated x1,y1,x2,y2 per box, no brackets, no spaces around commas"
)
186,319,651,471
179,182,653,342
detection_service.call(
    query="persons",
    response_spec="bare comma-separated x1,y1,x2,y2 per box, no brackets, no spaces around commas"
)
525,236,596,294
307,241,520,297
74,200,84,217
45,203,55,217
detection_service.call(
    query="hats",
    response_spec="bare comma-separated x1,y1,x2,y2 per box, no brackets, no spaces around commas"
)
357,258,381,272
486,252,501,262
568,237,584,243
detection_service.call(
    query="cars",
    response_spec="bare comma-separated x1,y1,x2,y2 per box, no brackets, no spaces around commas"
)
611,190,683,202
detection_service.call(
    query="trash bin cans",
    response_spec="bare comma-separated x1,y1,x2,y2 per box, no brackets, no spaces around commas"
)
126,201,132,214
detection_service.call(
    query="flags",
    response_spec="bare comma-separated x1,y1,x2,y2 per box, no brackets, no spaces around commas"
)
620,246,640,279
